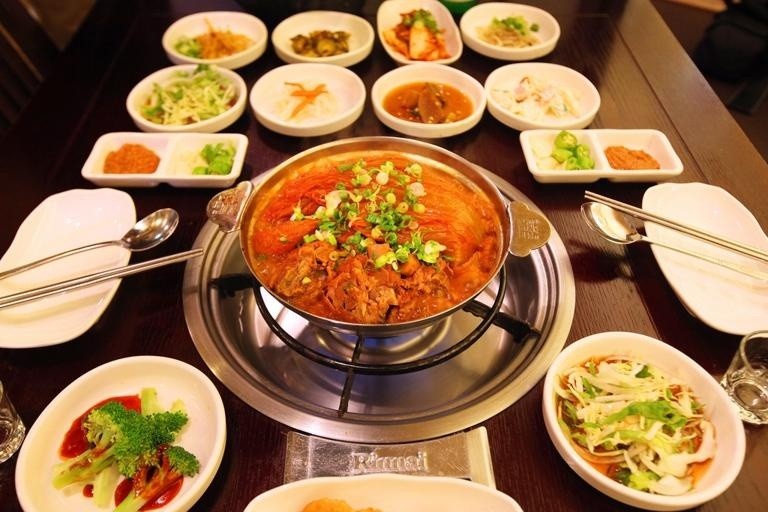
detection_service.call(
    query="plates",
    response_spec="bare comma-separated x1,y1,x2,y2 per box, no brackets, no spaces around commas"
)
371,62,488,140
481,62,602,133
271,10,375,67
79,131,249,188
542,331,747,511
1,189,137,349
14,353,226,512
249,63,367,137
642,182,768,337
375,0,465,65
127,66,248,136
246,473,524,512
519,127,685,183
458,3,561,62
160,11,267,71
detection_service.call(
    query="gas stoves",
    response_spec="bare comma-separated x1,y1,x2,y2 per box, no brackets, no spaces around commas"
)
183,159,576,443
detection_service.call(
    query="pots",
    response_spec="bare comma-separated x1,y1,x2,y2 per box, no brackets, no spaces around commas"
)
205,134,552,335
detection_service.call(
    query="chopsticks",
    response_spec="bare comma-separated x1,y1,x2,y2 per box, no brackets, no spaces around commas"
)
584,189,767,260
0,248,204,309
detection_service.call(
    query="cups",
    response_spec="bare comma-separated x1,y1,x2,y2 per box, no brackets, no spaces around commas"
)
0,381,25,464
720,329,768,426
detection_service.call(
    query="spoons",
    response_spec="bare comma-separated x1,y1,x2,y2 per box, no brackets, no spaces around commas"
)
0,208,180,280
580,202,767,280
